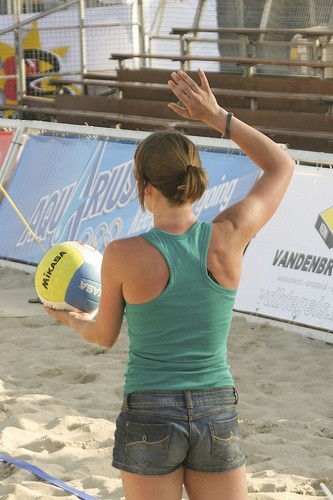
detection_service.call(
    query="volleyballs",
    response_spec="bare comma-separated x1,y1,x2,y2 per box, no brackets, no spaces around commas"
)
34,240,106,314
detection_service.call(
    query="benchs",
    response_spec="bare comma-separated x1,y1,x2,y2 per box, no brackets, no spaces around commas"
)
1,26,333,168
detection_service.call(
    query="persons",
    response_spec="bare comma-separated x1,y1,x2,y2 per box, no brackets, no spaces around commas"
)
42,65,296,499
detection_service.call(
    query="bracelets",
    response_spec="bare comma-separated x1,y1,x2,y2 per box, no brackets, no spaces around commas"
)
222,112,233,140
